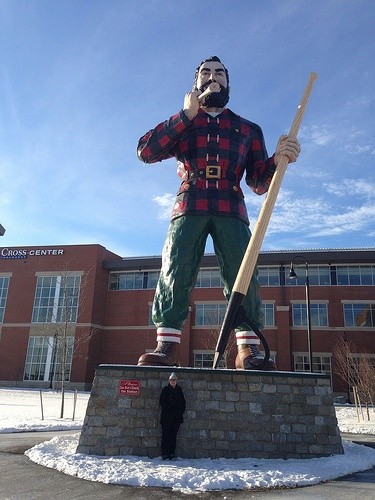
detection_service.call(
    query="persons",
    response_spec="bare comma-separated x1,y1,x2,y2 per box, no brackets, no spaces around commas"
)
159,372,187,460
137,56,301,369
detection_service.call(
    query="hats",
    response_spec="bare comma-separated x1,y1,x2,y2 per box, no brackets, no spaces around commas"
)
169,372,178,382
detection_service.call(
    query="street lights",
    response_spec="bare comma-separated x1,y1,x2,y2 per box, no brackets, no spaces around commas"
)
47,329,58,388
287,255,313,373
